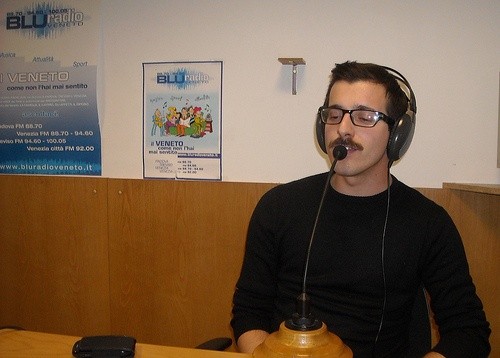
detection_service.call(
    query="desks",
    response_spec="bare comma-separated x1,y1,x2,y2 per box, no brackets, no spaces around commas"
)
0,326,254,358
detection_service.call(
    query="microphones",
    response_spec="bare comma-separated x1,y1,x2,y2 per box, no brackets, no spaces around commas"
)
297,145,347,318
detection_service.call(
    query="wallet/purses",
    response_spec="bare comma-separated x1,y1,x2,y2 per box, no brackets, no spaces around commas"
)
72,336,136,358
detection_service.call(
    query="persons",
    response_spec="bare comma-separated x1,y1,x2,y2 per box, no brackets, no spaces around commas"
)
230,60,492,358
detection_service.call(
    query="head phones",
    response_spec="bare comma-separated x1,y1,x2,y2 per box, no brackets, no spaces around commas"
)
316,67,417,161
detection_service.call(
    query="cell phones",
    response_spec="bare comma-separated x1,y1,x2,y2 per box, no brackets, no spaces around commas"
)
72,335,137,358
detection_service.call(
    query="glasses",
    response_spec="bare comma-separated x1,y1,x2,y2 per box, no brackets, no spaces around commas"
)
319,106,394,128
